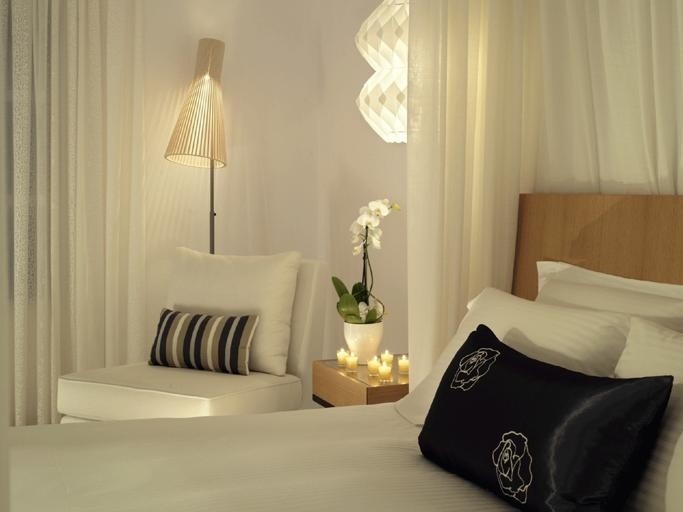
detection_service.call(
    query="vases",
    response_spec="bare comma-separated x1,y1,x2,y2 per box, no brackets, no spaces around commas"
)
344,320,384,365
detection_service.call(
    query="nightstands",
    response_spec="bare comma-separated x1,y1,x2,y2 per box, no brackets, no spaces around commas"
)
312,354,409,408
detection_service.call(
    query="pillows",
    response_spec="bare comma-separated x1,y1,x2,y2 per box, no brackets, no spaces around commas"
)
165,246,298,376
148,308,259,376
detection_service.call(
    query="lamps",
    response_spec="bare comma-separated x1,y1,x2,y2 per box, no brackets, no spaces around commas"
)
355,0,409,144
164,38,227,253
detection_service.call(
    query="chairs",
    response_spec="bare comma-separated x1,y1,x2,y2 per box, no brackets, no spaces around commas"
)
57,261,330,424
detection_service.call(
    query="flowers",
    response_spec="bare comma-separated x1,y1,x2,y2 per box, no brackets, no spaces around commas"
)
331,198,401,324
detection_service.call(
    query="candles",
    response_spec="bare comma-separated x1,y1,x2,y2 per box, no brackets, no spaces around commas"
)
336,345,410,383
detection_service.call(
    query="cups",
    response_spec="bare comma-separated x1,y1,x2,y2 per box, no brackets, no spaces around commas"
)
336,350,408,382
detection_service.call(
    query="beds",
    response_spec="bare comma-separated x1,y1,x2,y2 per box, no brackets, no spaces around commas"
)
0,193,683,512
